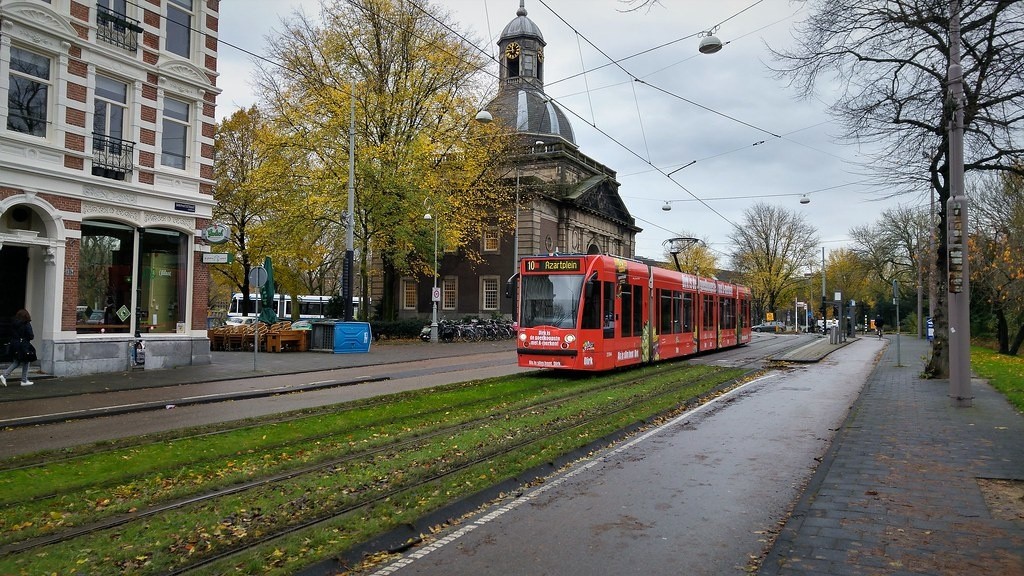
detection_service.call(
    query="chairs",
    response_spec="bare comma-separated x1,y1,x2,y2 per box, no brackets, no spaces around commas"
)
212,321,292,352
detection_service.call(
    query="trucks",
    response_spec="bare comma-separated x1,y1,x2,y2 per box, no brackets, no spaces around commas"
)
817,320,839,334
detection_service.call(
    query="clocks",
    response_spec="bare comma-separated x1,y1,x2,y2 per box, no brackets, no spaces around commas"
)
537,47,544,63
505,42,520,59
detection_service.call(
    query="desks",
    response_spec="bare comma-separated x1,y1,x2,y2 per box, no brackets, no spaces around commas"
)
267,330,307,352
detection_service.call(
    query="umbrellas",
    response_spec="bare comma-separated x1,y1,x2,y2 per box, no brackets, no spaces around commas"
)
257,253,278,329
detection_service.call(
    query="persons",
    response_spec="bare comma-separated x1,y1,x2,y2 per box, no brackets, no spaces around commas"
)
809,317,816,333
0,308,36,387
874,314,884,337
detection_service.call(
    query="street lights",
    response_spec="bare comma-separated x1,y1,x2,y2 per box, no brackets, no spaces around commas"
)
424,197,442,343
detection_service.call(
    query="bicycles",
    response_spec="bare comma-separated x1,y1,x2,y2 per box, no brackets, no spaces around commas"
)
874,324,883,340
420,314,517,342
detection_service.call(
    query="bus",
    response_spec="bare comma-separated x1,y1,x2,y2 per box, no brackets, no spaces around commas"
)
506,254,752,372
227,293,372,322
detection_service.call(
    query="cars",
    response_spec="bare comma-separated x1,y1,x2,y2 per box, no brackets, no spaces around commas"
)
290,319,323,331
225,317,257,327
752,322,786,333
77,311,105,325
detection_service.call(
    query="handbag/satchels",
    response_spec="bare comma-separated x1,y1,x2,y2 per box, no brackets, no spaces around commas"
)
19,341,37,363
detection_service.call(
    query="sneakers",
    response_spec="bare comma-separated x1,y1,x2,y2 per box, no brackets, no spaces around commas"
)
20,381,34,386
0,375,8,387
